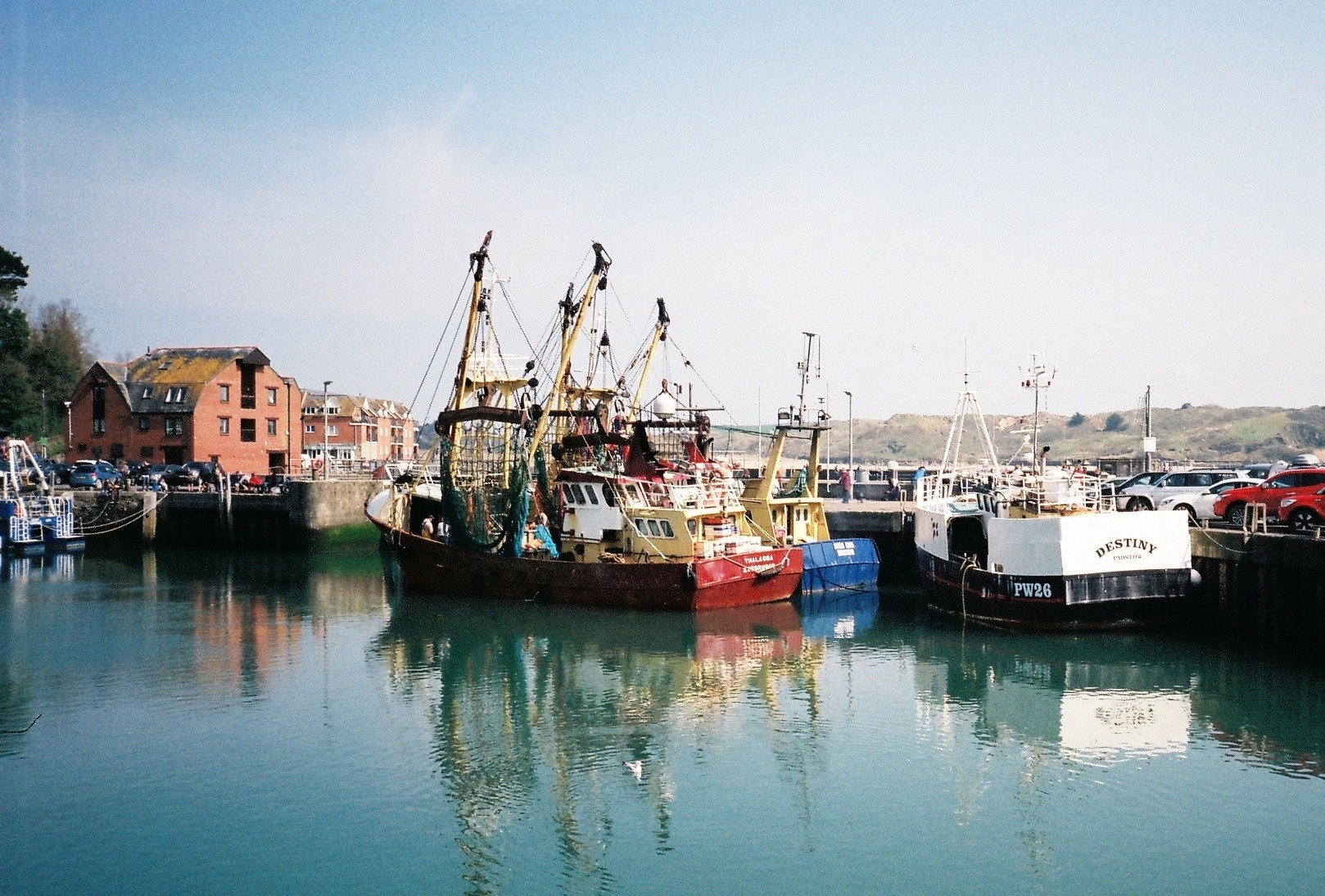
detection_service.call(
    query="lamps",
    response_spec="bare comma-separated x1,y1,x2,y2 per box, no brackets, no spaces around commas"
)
816,409,831,427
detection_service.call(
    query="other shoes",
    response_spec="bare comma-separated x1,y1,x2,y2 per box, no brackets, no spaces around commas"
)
842,501,849,504
262,490,271,494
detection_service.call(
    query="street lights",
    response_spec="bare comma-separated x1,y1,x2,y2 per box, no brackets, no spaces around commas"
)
322,379,333,479
841,390,852,472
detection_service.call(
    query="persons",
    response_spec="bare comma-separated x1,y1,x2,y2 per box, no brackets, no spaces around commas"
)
104,459,291,502
1013,465,1024,475
535,509,548,526
421,515,451,543
913,466,926,501
802,465,862,504
885,478,901,501
1075,460,1101,507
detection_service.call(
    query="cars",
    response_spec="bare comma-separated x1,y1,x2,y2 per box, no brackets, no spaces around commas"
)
0,457,227,488
1116,466,1324,530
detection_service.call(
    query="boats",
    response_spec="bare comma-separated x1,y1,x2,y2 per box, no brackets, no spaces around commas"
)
914,367,1195,629
362,229,806,617
0,436,78,547
739,330,879,597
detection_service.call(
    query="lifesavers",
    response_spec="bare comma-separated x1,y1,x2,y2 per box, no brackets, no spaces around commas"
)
703,516,730,524
647,483,665,507
312,459,324,472
709,469,725,478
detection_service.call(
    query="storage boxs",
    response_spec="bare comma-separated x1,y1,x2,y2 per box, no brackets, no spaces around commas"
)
694,516,774,559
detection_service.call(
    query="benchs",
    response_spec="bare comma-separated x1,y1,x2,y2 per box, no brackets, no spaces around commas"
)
231,474,284,492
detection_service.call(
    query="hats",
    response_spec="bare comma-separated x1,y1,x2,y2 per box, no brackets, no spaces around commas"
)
429,515,435,520
840,467,847,470
